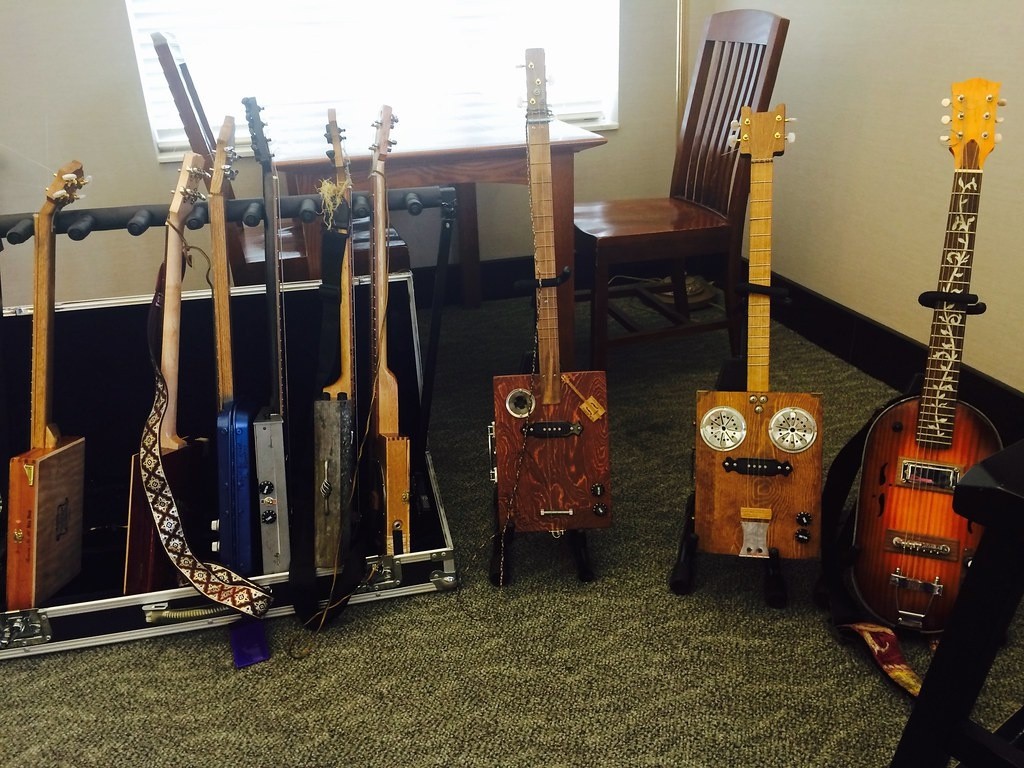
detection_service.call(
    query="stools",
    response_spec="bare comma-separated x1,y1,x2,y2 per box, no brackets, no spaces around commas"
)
893,437,1024,768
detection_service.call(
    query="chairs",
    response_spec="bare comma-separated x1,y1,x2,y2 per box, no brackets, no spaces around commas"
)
572,10,792,394
151,30,410,287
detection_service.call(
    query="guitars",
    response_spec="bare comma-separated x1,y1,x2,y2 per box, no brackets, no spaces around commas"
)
239,96,293,574
695,105,823,561
492,48,613,533
5,159,94,611
205,116,254,580
369,105,411,555
847,77,1005,631
310,107,356,576
123,151,211,597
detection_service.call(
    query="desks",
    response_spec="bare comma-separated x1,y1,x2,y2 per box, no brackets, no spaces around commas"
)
272,116,608,380
